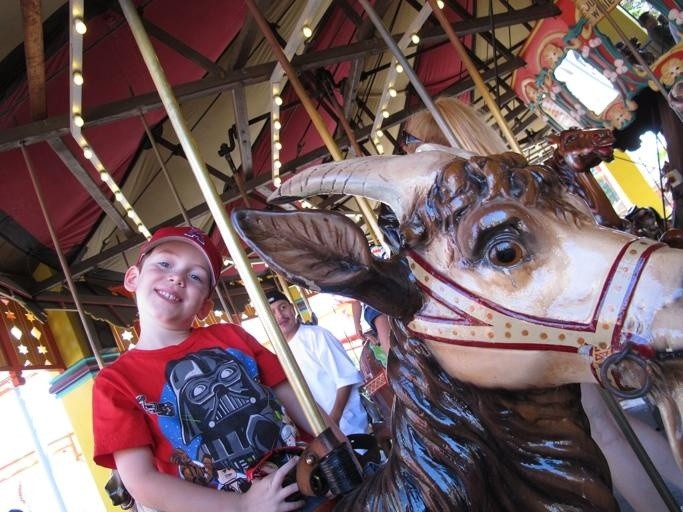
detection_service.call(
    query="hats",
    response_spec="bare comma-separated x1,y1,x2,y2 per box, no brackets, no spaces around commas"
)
135,225,224,300
264,290,290,308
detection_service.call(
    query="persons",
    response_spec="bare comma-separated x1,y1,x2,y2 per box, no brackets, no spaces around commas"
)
608,2,682,64
400,95,507,165
262,288,370,439
92,225,362,512
364,303,390,355
